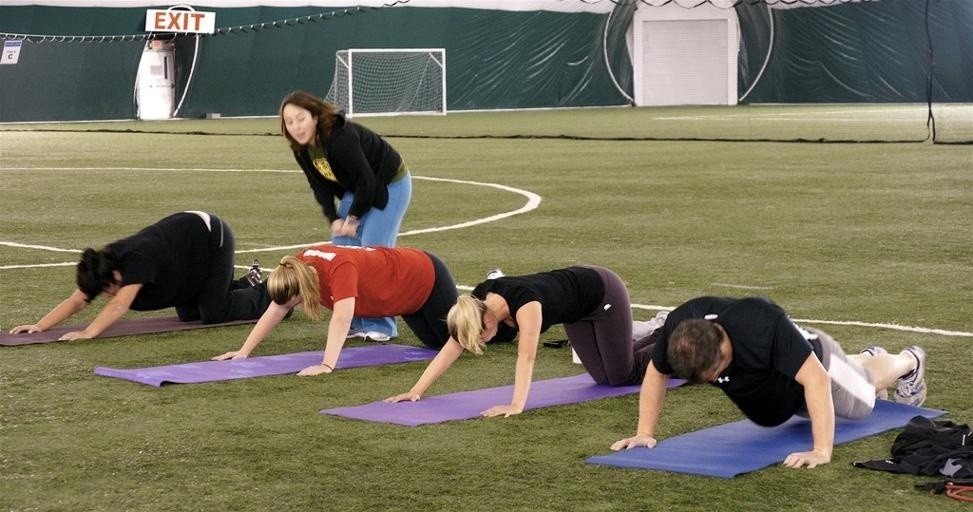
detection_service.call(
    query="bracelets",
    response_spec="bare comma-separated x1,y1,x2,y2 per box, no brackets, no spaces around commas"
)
320,362,333,372
346,215,361,227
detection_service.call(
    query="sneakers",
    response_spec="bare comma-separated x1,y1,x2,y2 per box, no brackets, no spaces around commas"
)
861,347,888,401
347,331,391,341
895,346,927,406
243,267,263,286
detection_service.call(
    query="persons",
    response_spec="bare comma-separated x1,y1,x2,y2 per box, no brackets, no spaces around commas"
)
211,245,517,378
610,295,927,469
10,211,294,342
382,264,665,419
279,91,412,249
